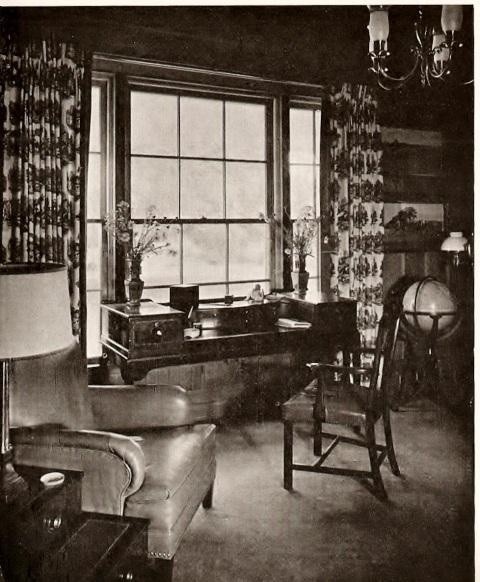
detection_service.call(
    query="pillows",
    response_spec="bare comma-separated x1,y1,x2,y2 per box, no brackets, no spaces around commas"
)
440,231,468,273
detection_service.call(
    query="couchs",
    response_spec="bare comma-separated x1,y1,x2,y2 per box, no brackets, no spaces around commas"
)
3,344,218,573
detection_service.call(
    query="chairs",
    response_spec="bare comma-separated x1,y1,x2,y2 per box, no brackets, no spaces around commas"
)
281,305,401,503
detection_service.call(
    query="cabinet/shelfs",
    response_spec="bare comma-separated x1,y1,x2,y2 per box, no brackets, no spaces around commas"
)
0,464,173,582
99,291,361,385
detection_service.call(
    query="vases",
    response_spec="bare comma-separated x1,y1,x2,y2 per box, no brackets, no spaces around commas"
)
124,259,144,308
290,261,309,295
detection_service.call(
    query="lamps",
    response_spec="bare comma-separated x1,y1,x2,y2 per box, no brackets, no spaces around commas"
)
367,5,474,91
0,261,77,504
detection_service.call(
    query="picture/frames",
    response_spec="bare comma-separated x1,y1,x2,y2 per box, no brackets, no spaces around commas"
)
383,200,452,254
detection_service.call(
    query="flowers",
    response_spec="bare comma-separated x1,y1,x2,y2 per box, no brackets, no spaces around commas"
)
102,200,178,259
259,205,329,261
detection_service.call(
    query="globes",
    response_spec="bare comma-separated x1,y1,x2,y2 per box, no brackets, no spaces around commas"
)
391,275,465,411
